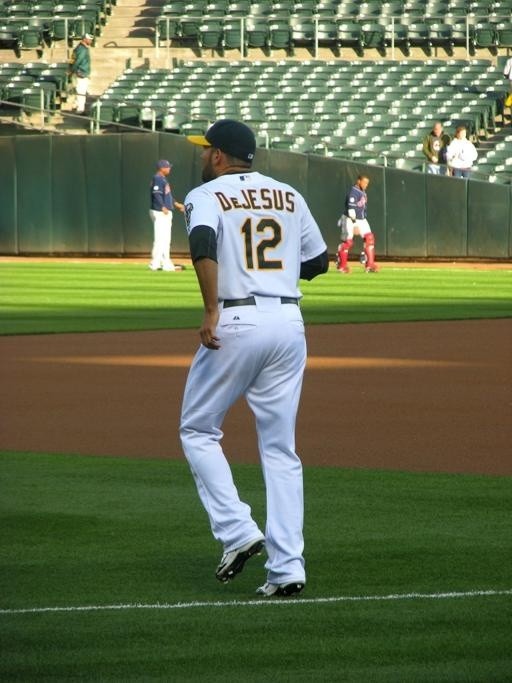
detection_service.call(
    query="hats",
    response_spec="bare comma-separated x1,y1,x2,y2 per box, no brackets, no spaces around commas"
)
84,33,94,40
187,119,256,162
156,159,173,170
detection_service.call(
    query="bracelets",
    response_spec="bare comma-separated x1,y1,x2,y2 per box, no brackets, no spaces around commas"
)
351,217,357,223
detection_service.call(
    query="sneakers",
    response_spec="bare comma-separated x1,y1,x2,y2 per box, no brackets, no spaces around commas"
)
256,581,306,597
364,264,379,272
149,264,175,272
215,534,265,583
337,266,350,273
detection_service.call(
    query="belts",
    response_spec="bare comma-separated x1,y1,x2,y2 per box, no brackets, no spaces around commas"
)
223,294,298,308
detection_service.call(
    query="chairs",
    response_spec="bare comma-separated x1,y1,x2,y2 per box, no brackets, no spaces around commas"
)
0,0,511,189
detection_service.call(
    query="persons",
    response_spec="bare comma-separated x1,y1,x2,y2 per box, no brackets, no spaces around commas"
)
503,55,512,96
334,172,381,273
148,158,186,272
178,118,330,599
444,125,478,177
422,120,452,176
64,32,94,116
